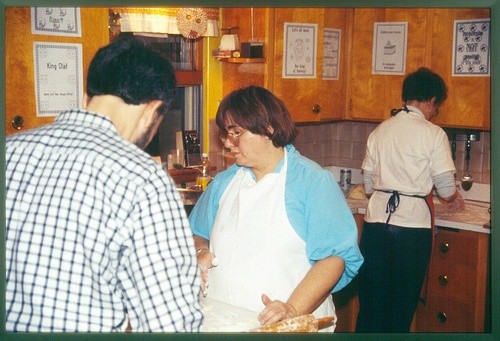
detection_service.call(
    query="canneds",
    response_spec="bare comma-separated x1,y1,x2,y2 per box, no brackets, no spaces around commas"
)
340,169,352,191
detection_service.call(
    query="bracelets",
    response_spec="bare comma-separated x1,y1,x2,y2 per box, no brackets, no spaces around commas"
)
197,248,207,255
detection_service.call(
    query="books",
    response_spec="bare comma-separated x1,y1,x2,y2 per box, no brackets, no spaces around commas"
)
158,36,200,131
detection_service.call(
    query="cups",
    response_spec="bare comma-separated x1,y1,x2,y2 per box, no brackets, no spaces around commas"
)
340,170,351,191
170,149,185,170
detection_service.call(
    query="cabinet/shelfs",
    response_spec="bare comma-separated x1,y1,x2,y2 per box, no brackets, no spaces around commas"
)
221,8,491,131
330,213,492,333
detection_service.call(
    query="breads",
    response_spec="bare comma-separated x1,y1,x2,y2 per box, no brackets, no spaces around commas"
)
348,183,368,200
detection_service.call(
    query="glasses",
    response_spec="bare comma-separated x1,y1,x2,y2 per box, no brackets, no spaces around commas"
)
219,129,248,146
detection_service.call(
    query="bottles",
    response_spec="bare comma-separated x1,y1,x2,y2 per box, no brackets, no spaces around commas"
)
196,153,212,192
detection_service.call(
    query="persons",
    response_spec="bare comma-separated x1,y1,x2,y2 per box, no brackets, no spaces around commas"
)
354,68,459,333
4,38,203,335
186,86,365,334
